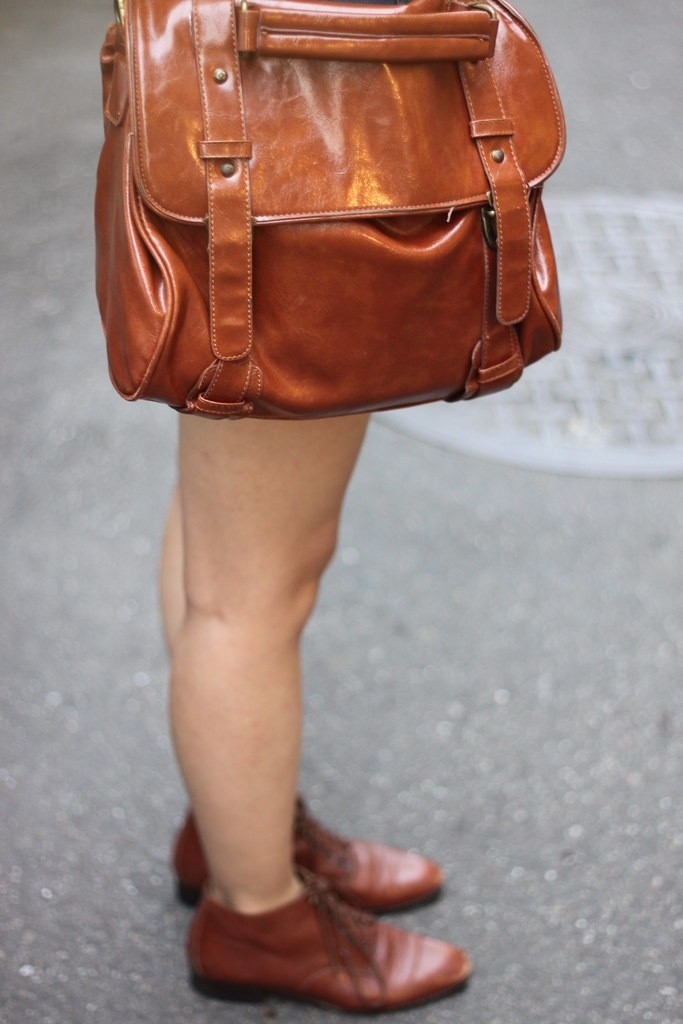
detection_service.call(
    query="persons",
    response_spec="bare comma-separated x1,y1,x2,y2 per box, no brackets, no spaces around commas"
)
160,1,471,1014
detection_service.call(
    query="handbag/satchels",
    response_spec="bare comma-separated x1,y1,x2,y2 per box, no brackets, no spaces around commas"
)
88,1,564,423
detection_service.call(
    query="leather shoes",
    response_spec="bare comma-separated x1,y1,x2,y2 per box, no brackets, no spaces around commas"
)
168,790,445,916
188,863,472,1014
